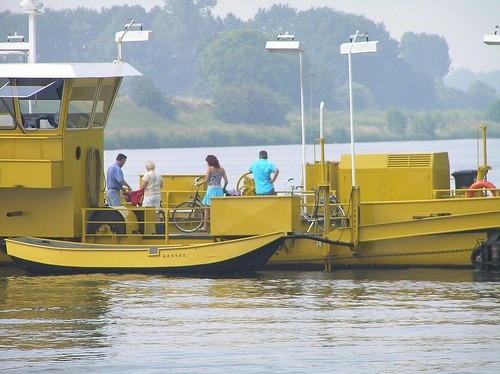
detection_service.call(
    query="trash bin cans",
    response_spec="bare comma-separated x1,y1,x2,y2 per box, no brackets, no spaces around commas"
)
451,170,478,188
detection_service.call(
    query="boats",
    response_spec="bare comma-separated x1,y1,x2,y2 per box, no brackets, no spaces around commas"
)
0,0,500,278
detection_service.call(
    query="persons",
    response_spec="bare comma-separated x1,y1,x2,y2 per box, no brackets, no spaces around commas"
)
248,151,279,196
141,161,163,222
106,153,132,206
194,155,228,232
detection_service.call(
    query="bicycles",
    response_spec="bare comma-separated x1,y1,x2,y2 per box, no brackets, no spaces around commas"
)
287,176,347,233
173,176,241,233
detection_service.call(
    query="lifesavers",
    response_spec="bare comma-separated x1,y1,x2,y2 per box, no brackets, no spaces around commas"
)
467,181,496,197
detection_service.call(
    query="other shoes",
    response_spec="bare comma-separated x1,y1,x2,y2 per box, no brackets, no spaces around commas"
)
198,228,209,231
